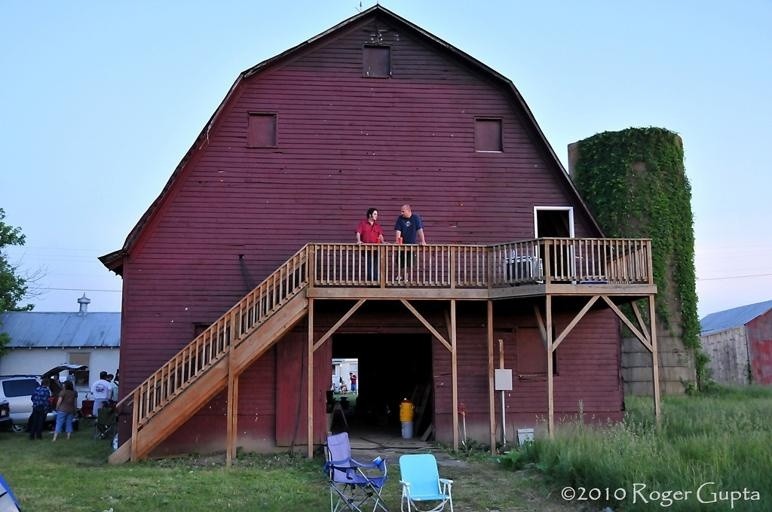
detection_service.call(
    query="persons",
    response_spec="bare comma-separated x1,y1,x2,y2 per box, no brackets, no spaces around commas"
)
331,381,336,392
349,372,357,395
392,204,427,281
30,378,51,440
90,370,114,434
354,207,391,284
338,379,348,394
52,380,78,442
104,374,115,398
113,369,119,403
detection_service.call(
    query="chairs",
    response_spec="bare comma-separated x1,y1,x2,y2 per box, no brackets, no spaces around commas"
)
321,431,454,511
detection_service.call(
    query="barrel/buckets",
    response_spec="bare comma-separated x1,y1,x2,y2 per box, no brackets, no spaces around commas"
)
401,422,413,439
399,400,413,422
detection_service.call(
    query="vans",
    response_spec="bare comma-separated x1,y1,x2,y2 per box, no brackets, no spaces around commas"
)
0,364,88,433
